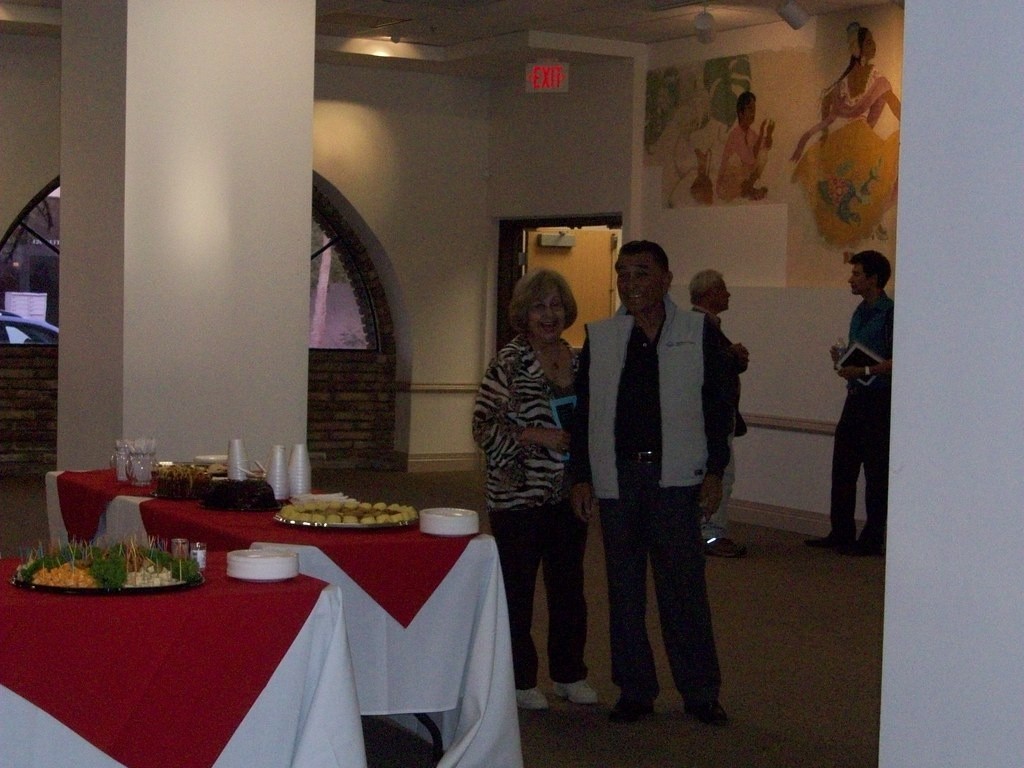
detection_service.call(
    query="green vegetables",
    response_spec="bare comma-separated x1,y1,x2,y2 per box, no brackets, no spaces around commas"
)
21,543,199,587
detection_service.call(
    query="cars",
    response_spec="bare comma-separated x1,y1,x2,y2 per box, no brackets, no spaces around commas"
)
0,309,58,344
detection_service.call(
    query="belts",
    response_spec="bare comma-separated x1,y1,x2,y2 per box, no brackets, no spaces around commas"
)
617,452,662,465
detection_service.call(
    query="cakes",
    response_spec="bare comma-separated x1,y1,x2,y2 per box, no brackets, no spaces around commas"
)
151,464,228,496
203,479,276,508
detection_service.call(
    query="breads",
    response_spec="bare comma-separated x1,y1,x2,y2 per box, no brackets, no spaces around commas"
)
279,499,417,523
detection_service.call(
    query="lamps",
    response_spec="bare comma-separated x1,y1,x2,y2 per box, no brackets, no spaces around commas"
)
693,0,716,44
776,0,809,30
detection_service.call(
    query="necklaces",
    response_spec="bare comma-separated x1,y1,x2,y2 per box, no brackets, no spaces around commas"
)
553,362,558,367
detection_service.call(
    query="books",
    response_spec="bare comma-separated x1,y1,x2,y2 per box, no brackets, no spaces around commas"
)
837,342,883,386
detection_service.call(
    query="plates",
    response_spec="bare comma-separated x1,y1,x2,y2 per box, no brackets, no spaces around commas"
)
8,572,206,596
419,507,480,538
226,548,299,583
193,455,227,463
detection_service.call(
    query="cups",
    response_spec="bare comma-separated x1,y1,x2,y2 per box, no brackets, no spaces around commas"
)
265,443,311,500
110,446,130,482
228,439,248,480
125,451,156,486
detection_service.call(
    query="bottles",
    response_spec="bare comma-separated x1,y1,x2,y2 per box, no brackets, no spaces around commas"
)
190,543,207,572
833,337,846,370
171,538,189,560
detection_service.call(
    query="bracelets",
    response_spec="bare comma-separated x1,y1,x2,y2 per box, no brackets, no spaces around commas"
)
865,366,869,375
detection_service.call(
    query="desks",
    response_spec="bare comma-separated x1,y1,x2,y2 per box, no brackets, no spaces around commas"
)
0,552,368,768
48,465,523,768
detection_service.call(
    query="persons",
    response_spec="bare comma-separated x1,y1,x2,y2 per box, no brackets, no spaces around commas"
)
472,270,599,711
567,240,725,726
803,250,895,557
688,270,750,558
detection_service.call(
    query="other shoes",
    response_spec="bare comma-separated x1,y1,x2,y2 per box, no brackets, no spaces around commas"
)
552,680,598,703
703,536,739,557
684,699,727,725
609,695,654,723
836,539,884,557
805,530,857,550
516,686,549,709
